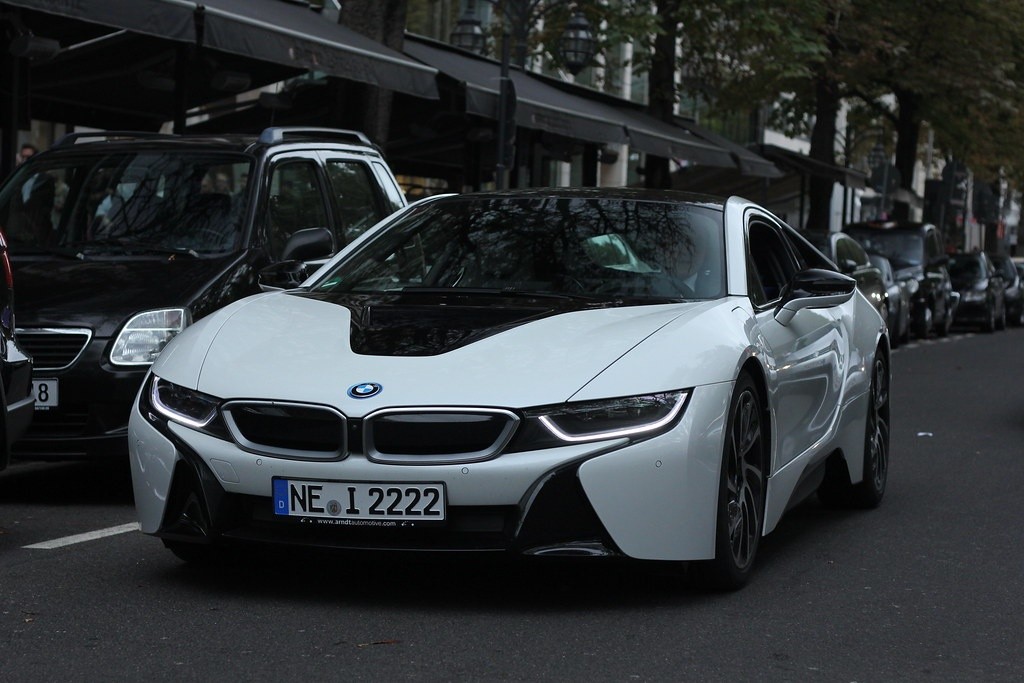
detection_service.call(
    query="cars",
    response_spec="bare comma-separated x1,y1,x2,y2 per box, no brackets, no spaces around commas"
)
949,251,1023,332
791,228,890,339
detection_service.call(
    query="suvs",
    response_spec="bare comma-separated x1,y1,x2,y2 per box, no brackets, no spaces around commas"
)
842,220,954,339
1,125,414,470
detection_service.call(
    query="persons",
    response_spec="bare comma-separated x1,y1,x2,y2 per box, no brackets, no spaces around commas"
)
586,213,719,296
11,143,384,250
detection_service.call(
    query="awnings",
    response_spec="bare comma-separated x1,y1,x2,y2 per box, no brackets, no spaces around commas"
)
765,144,928,209
0,0,786,179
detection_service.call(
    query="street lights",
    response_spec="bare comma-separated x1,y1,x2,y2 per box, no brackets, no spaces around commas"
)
833,124,888,233
446,0,605,190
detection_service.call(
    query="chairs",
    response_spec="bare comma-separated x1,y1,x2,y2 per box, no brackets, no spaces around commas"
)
171,193,230,247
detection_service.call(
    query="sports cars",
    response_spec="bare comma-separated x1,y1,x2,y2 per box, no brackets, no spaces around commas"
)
127,186,892,592
